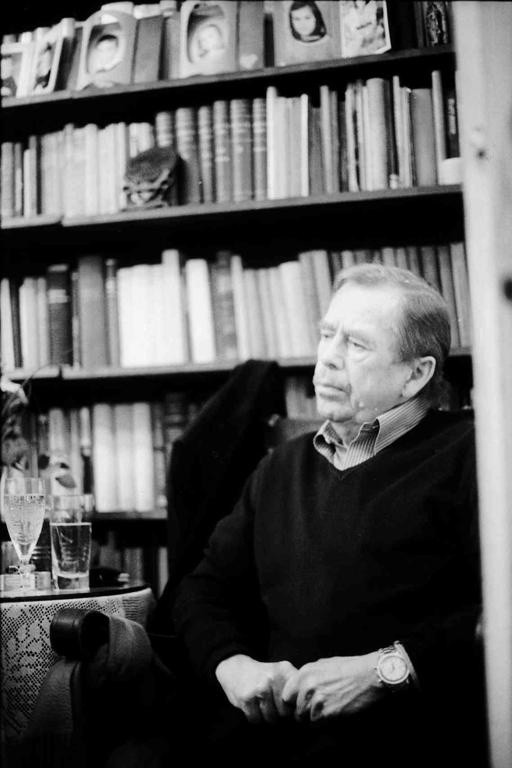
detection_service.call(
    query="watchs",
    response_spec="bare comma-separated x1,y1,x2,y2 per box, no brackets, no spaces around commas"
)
375,645,412,694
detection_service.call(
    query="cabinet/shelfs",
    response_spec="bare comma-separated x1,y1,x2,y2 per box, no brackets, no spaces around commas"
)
1,40,469,605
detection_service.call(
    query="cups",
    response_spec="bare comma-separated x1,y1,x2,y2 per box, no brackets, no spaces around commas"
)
48,492,93,589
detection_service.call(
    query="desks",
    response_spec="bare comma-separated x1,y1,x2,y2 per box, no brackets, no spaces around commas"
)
0,556,155,766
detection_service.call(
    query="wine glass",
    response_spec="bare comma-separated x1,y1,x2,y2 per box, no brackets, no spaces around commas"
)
4,477,47,589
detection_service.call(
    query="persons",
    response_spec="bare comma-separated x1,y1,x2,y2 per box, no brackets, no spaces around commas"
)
105,263,492,766
